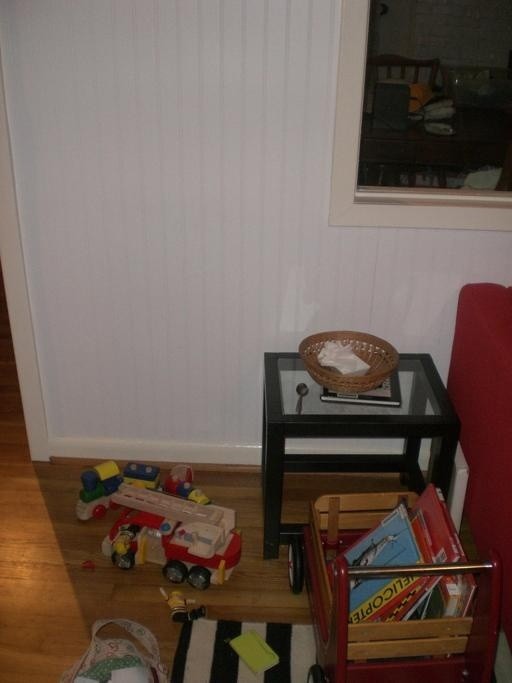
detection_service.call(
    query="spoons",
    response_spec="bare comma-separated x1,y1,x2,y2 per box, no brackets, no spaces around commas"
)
294,382,309,416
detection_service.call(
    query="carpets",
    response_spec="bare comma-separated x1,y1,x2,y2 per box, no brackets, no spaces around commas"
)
168,619,317,683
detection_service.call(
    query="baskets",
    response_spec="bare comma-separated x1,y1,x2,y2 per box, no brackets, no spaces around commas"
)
298,330,403,396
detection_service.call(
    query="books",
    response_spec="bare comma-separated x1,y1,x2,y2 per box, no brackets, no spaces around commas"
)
320,367,402,407
327,482,480,623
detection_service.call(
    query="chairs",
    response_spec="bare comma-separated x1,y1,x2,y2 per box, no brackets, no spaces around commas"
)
447,283,512,655
365,54,441,113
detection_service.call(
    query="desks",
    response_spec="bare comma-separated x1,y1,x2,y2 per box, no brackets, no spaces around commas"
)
359,101,512,191
262,350,459,560
